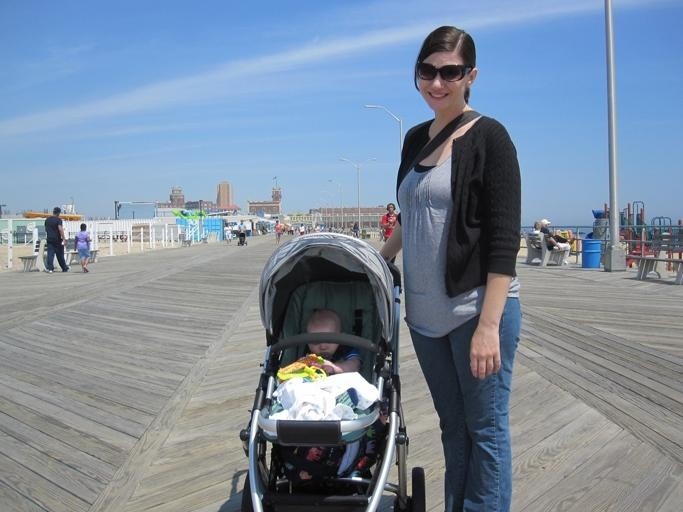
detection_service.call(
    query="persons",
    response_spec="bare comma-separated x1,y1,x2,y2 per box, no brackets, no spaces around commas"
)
238,220,246,233
74,223,90,273
379,203,399,264
44,207,71,272
540,219,571,265
534,221,575,249
303,308,361,409
273,220,361,244
377,25,523,512
224,223,233,245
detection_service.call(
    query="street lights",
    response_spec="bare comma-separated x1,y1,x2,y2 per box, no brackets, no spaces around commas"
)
310,153,379,230
361,103,403,151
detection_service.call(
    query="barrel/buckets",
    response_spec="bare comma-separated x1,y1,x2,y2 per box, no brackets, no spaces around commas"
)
583,237,601,248
581,251,602,268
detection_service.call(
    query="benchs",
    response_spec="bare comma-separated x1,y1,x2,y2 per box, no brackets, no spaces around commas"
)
179,234,191,247
18,238,100,272
362,230,371,239
523,232,570,267
630,228,683,285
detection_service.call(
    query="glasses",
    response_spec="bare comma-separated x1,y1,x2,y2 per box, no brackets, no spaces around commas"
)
417,63,472,82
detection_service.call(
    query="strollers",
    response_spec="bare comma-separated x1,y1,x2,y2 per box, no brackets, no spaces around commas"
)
211,228,428,511
237,232,248,247
